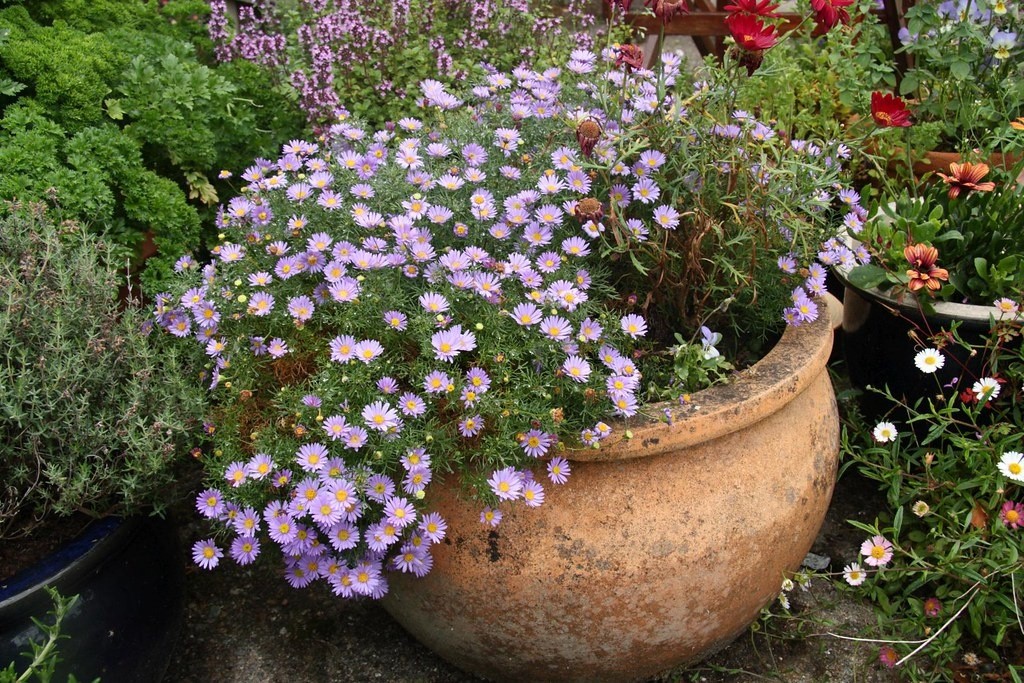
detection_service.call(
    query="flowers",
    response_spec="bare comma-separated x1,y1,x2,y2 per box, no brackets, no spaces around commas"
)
149,47,873,619
846,153,1023,315
892,0,1024,155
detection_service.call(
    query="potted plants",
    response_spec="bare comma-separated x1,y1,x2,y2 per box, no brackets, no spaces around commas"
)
1,187,237,683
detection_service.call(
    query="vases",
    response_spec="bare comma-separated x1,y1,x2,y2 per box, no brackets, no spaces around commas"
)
846,96,1024,198
377,291,845,683
846,261,1023,447
